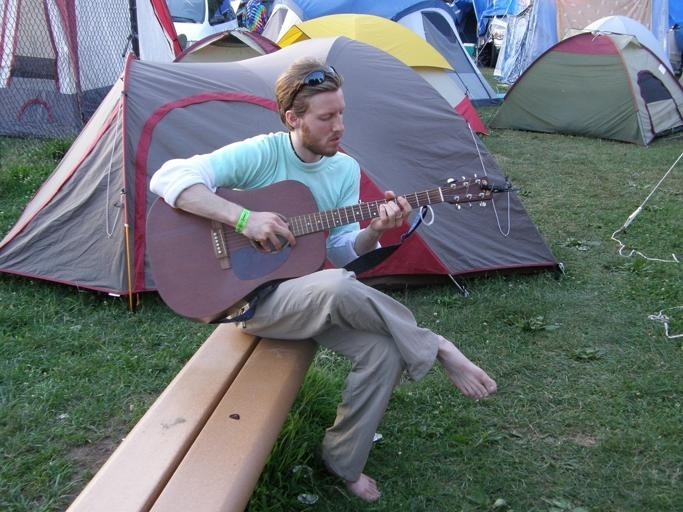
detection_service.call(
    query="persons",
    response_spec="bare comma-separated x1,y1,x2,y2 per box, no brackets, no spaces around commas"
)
148,58,499,503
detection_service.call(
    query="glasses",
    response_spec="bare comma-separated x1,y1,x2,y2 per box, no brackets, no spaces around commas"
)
285,65,337,112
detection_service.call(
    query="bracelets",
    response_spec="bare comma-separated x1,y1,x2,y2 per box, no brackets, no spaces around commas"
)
234,207,250,234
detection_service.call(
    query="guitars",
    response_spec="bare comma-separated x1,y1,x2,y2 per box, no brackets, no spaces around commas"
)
146,174,493,324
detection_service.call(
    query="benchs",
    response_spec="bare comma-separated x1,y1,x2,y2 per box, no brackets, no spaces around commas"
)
64,323,318,512
